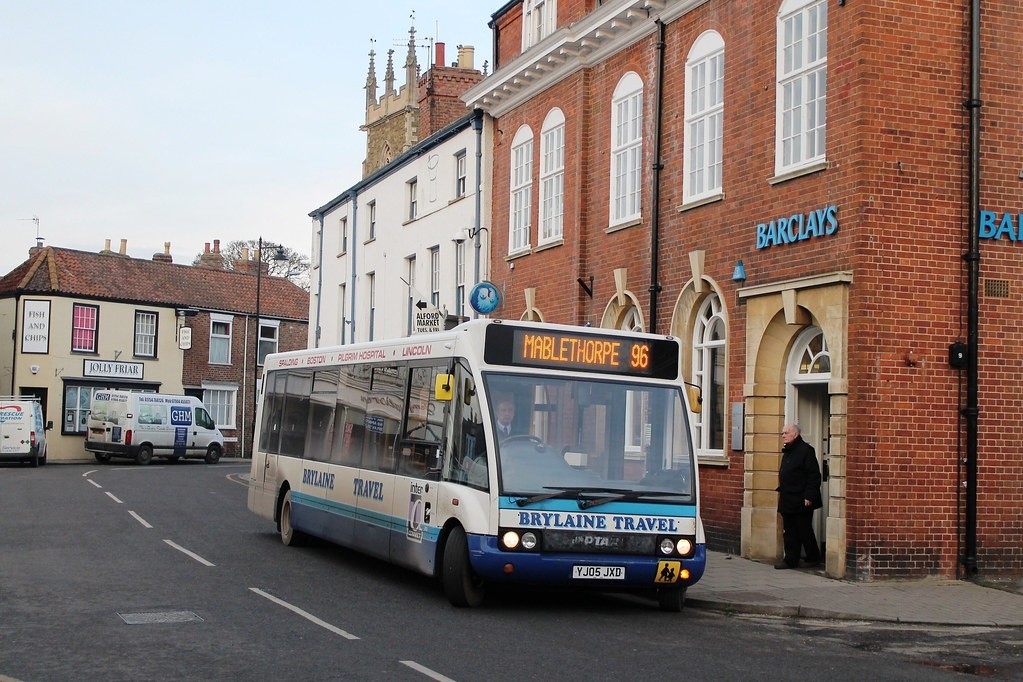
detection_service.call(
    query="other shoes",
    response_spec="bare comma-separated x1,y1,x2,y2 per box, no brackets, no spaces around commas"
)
799,561,820,568
774,560,800,569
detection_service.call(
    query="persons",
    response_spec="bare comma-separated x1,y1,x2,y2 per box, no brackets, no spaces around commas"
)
773,422,824,569
474,398,530,457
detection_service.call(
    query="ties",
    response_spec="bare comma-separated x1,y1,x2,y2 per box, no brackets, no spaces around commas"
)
504,427,508,439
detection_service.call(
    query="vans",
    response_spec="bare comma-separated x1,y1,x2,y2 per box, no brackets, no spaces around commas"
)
84,390,225,463
0,393,54,466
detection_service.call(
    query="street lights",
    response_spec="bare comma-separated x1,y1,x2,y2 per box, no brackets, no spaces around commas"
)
250,238,287,455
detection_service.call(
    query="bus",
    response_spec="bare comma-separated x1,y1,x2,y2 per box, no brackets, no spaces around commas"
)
246,319,708,608
